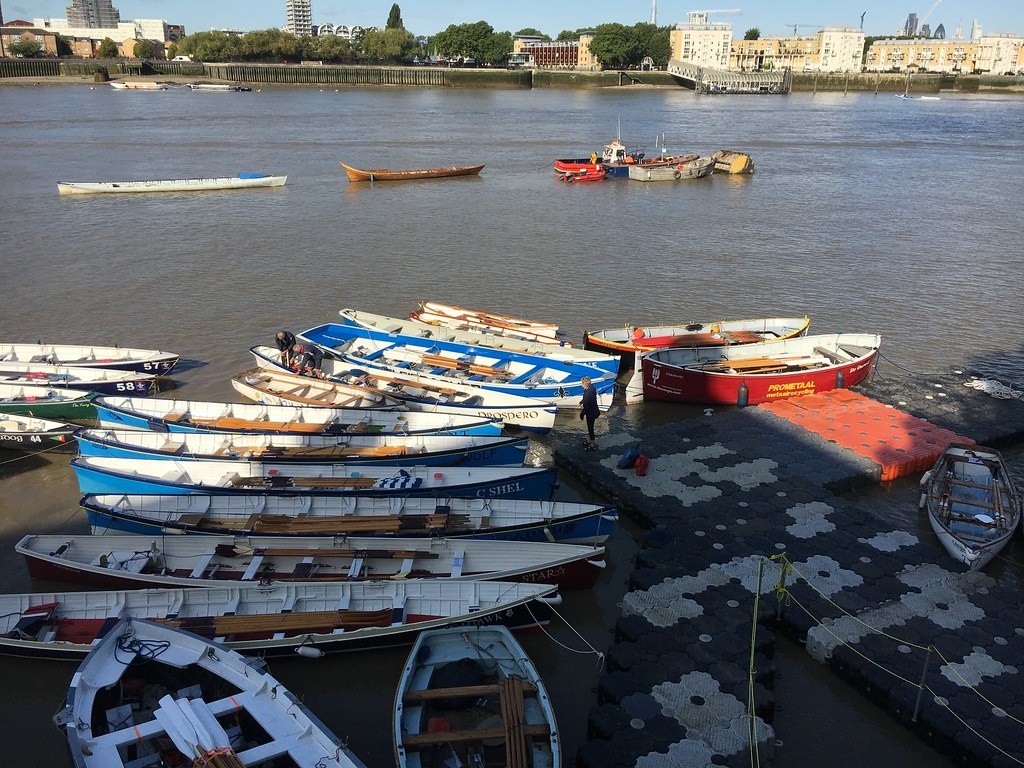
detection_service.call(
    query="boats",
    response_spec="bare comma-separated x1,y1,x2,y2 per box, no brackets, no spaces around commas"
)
392,623,562,768
581,313,812,370
185,84,238,92
339,161,487,183
52,614,367,768
0,577,559,662
110,81,169,90
665,58,793,95
15,527,606,594
0,293,619,554
920,441,1021,571
551,119,718,182
56,174,288,196
635,332,883,407
895,94,940,101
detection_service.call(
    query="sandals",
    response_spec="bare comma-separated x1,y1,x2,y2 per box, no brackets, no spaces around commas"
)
581,440,593,445
584,445,597,451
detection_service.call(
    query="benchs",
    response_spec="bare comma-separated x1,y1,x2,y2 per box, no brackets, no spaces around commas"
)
468,596,480,613
165,601,184,619
120,551,149,573
54,540,71,556
241,556,263,579
110,695,244,747
189,550,215,578
780,343,1015,543
390,596,407,626
236,740,287,767
272,599,295,639
400,559,414,572
348,558,363,577
0,314,553,531
91,606,125,645
292,557,314,578
213,600,240,642
332,597,351,634
451,551,465,577
403,681,537,708
403,724,549,753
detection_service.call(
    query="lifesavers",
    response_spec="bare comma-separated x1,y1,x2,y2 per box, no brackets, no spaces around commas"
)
696,169,704,177
673,171,682,179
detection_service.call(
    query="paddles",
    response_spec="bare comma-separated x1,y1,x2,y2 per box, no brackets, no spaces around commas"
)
260,572,432,581
496,672,528,768
249,512,478,531
227,444,415,459
675,328,767,343
349,367,469,395
173,421,388,432
937,455,958,522
212,541,445,561
695,354,827,373
153,692,245,768
989,457,1007,531
232,475,425,490
148,606,396,636
421,352,517,380
229,548,439,558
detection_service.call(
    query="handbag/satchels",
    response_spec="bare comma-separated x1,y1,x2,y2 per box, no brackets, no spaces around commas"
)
592,405,600,419
635,456,649,476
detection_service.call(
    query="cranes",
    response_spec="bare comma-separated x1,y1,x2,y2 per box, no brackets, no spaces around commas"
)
786,23,821,37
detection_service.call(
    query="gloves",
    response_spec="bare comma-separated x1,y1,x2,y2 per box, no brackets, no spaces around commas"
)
580,413,584,420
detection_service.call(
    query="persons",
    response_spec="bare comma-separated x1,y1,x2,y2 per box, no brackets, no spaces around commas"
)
603,146,611,163
590,151,598,164
275,331,296,368
575,376,601,451
289,343,323,379
618,154,635,164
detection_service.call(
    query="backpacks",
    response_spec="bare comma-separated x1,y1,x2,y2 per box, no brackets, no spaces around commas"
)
616,443,641,469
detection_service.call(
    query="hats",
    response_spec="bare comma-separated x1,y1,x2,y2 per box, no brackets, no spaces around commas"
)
293,344,301,351
278,332,285,340
